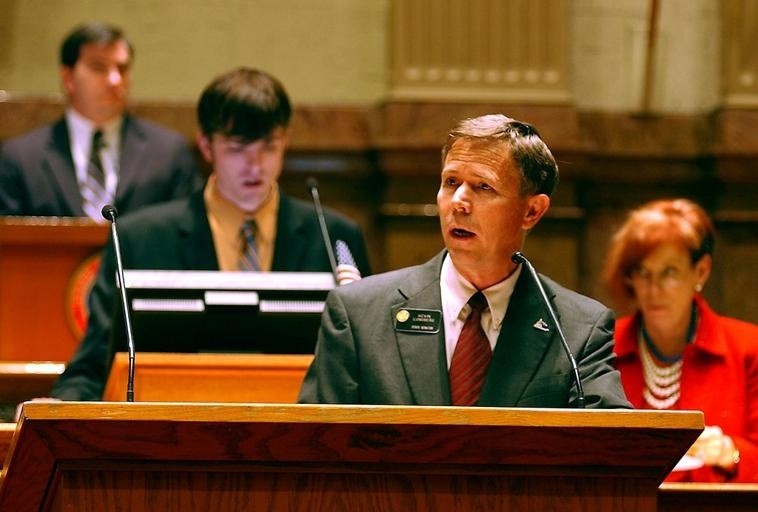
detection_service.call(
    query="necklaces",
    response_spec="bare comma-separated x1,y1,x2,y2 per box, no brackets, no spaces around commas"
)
636,299,701,410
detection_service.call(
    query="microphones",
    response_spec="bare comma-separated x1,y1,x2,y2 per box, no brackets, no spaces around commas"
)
306,176,340,287
101,203,134,401
511,250,585,408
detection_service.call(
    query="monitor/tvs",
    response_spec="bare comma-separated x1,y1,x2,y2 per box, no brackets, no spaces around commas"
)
109,268,336,369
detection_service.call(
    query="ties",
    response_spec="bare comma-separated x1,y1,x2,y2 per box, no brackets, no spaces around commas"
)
80,129,110,216
237,220,262,272
448,293,493,407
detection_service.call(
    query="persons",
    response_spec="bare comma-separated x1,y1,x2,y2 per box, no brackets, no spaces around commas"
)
0,23,201,219
298,113,631,407
33,69,373,403
606,197,757,483
